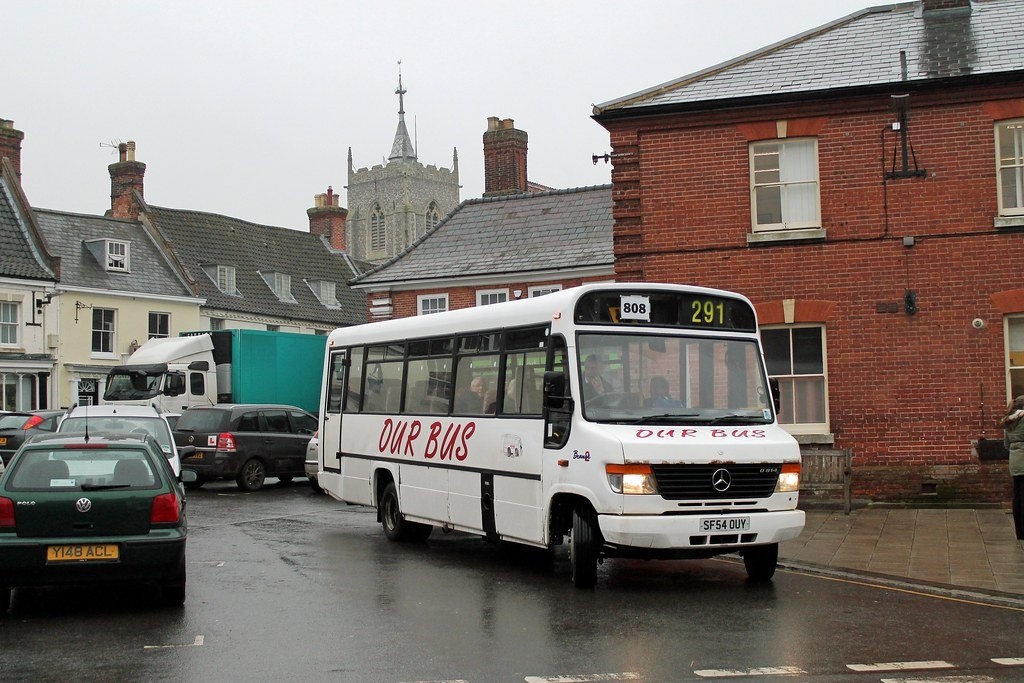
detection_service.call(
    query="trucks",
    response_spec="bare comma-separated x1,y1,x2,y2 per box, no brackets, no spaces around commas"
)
100,326,328,420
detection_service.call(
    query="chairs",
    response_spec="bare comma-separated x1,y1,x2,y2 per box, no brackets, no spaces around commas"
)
108,459,154,485
368,365,544,412
29,460,69,486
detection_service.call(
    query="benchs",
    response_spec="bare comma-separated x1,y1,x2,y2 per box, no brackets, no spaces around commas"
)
799,448,852,516
346,377,402,414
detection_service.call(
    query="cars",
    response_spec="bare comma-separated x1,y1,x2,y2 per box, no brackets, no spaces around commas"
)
0,409,68,478
0,431,188,618
303,428,324,494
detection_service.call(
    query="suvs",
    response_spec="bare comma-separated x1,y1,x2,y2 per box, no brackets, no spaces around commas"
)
46,402,186,497
173,402,319,493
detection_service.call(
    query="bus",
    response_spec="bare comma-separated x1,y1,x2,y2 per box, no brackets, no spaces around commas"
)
316,280,808,592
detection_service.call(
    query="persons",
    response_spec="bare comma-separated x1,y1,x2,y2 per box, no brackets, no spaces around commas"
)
998,396,1024,550
458,377,486,414
502,378,517,412
566,354,616,408
642,377,671,408
